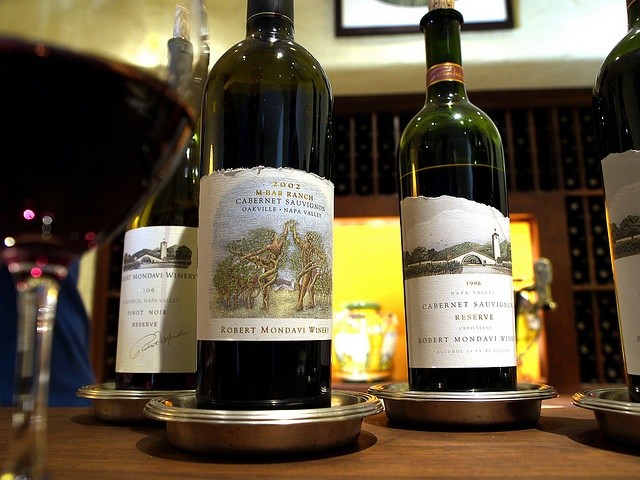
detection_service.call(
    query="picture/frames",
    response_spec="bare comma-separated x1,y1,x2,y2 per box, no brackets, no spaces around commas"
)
335,0,514,38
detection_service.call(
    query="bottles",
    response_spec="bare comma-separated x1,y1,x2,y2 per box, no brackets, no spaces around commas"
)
196,1,336,409
591,0,640,403
111,4,201,391
396,0,518,391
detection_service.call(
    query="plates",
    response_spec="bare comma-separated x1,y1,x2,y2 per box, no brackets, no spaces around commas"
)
571,387,640,442
143,388,385,456
366,381,558,428
76,381,197,422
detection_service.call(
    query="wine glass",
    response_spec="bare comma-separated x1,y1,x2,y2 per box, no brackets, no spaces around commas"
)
0,0,211,480
